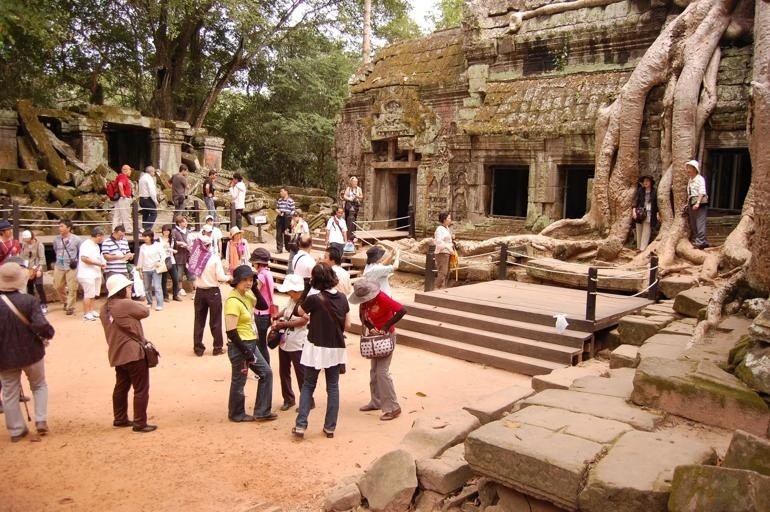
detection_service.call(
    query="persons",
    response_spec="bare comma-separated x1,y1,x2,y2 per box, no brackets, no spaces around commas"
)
682,160,711,247
0,163,401,443
433,210,454,291
632,174,660,255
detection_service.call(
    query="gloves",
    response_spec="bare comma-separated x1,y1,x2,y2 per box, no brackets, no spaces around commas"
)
226,328,257,365
251,274,269,311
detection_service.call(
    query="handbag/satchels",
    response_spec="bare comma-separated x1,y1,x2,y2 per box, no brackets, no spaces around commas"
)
69,260,78,269
127,263,136,273
360,334,394,359
154,257,172,274
267,304,287,349
450,250,458,268
288,239,300,252
689,195,708,206
28,268,36,279
632,207,647,224
107,180,120,201
142,340,160,368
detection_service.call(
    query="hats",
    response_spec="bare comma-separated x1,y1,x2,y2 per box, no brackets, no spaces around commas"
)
23,230,32,242
230,226,244,236
685,160,700,173
229,247,305,293
0,220,15,230
366,246,386,264
0,261,26,290
203,215,214,245
348,279,381,304
638,175,655,186
106,274,135,298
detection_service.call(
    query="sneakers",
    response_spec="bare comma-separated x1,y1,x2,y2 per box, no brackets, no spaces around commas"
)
41,305,48,316
380,408,401,420
67,309,73,315
113,421,133,427
360,406,372,411
241,402,334,438
173,294,183,301
37,422,49,435
214,349,226,355
134,426,157,432
155,306,162,311
90,311,99,318
83,313,96,321
11,427,29,443
